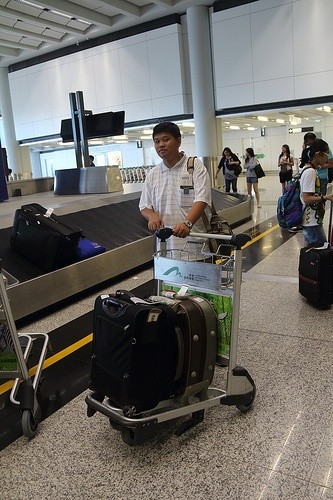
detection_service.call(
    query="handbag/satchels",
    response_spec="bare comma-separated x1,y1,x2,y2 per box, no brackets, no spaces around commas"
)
226,164,236,170
234,165,242,177
9,202,81,273
279,169,292,183
201,203,234,259
253,157,265,178
77,237,106,258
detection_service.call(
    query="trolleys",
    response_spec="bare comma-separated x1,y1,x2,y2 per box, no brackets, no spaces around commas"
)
0,268,49,438
85,226,257,447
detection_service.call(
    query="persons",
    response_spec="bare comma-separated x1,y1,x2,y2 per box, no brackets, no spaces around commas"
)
7,169,12,181
89,155,96,167
137,122,213,265
243,148,262,208
278,144,293,195
294,138,330,250
301,132,333,204
216,147,242,193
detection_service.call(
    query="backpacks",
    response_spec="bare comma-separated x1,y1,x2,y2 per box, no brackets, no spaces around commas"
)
277,167,313,228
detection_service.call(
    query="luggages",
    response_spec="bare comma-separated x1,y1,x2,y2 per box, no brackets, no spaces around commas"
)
299,242,333,307
87,289,190,414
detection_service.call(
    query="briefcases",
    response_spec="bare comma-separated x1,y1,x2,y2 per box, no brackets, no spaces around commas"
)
147,285,218,408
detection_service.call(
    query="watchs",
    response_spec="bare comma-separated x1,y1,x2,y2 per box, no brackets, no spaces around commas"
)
182,220,193,229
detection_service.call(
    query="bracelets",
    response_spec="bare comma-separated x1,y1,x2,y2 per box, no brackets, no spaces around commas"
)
320,194,326,203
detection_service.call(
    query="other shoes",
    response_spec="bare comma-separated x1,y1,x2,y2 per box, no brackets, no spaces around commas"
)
288,228,303,233
257,204,261,208
